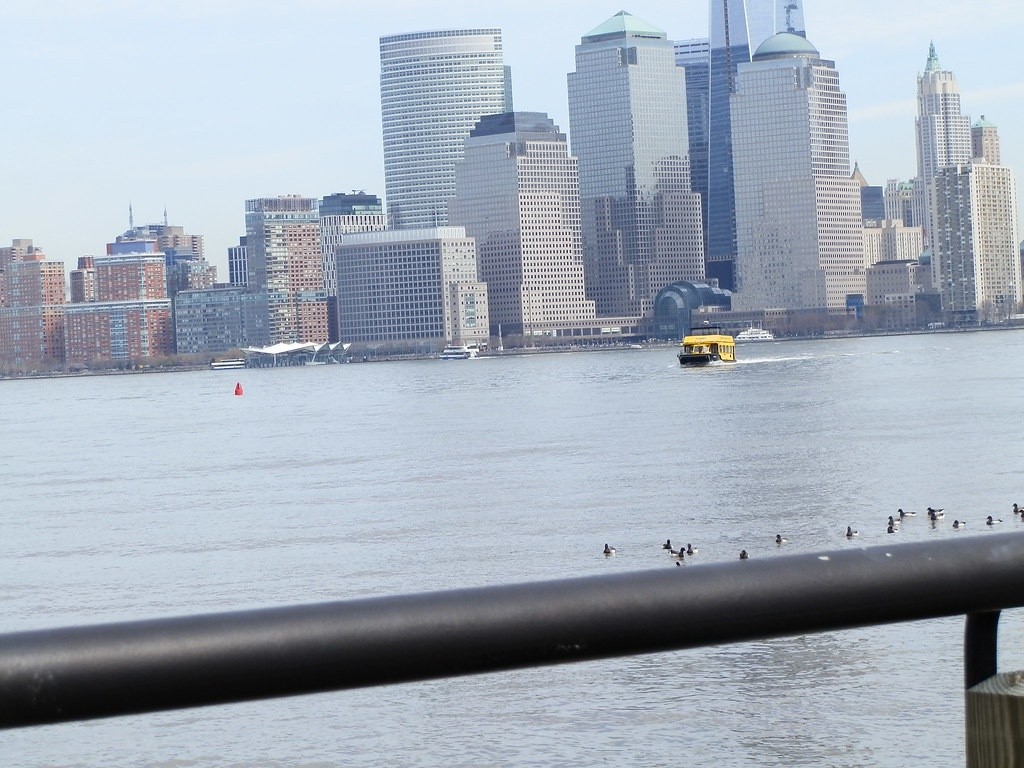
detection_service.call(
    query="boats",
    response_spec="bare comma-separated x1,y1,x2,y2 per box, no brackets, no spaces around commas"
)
438,345,479,359
734,327,774,343
676,335,737,367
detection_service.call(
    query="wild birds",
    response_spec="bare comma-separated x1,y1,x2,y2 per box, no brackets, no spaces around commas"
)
602,502,1023,566
